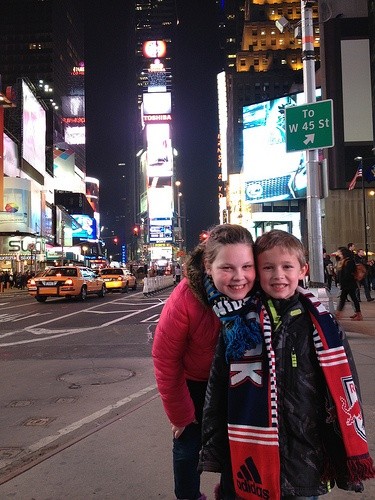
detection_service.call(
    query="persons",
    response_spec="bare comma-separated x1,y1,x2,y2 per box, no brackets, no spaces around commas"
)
344,242,357,254
198,228,374,500
331,247,366,321
322,247,333,292
150,223,258,500
0,265,129,294
355,249,375,302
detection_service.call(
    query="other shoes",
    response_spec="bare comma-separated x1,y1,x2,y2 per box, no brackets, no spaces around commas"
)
367,297,375,302
350,312,363,321
358,300,361,302
346,299,350,302
175,492,207,500
334,311,343,318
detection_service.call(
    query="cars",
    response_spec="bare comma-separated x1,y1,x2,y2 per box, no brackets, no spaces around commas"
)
27,264,107,302
98,267,138,294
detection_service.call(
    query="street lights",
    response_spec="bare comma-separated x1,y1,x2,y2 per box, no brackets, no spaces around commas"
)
176,180,182,248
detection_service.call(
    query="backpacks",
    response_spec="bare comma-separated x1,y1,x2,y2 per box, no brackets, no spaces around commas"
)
354,263,367,281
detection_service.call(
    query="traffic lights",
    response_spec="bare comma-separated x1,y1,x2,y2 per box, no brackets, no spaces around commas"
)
112,236,118,245
134,225,139,235
